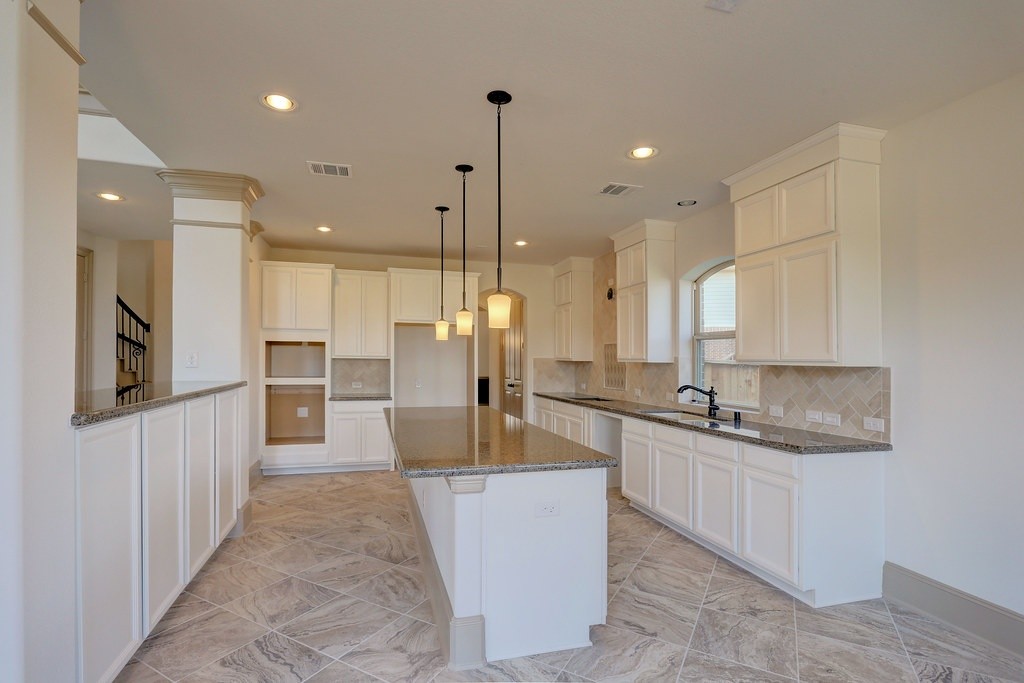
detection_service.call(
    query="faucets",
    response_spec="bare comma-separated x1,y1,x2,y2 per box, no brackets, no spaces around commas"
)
677,385,720,415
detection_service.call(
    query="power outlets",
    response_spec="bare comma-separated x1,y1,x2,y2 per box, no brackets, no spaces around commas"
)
634,388,640,396
768,405,784,418
665,392,674,402
862,416,884,433
580,383,586,389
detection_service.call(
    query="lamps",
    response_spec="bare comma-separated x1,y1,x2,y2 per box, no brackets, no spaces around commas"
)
456,164,478,341
486,88,514,331
435,204,450,343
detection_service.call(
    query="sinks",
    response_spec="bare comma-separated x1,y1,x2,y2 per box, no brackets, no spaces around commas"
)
647,412,726,421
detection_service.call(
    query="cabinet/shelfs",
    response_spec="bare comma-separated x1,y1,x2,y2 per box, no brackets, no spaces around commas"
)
261,260,330,463
187,389,242,595
332,400,392,463
80,401,184,683
618,416,694,540
552,401,590,445
615,219,680,366
381,403,623,670
332,268,391,360
386,265,482,327
720,121,887,372
553,257,594,362
693,433,894,607
533,395,553,431
